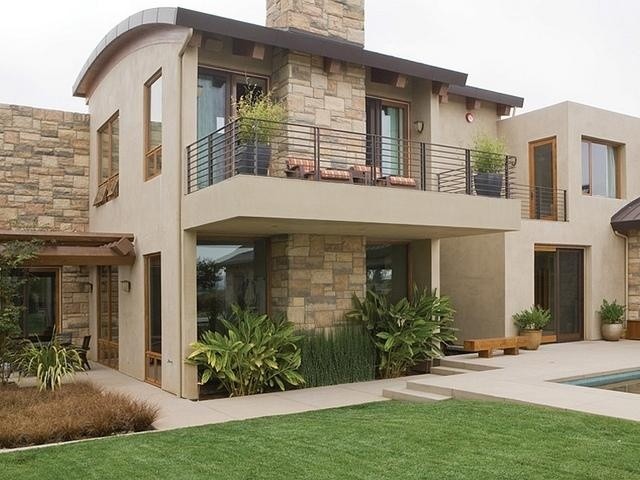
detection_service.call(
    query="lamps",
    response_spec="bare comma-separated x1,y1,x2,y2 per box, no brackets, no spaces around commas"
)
413,120,424,133
119,279,132,294
87,283,93,296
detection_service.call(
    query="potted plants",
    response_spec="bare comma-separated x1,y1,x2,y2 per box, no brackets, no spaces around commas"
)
470,131,508,199
511,303,553,351
594,298,630,343
226,71,291,175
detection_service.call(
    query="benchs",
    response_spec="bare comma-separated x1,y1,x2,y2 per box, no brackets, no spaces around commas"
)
462,335,531,359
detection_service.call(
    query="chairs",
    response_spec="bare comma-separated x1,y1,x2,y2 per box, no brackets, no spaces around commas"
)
6,331,92,372
287,156,418,189
